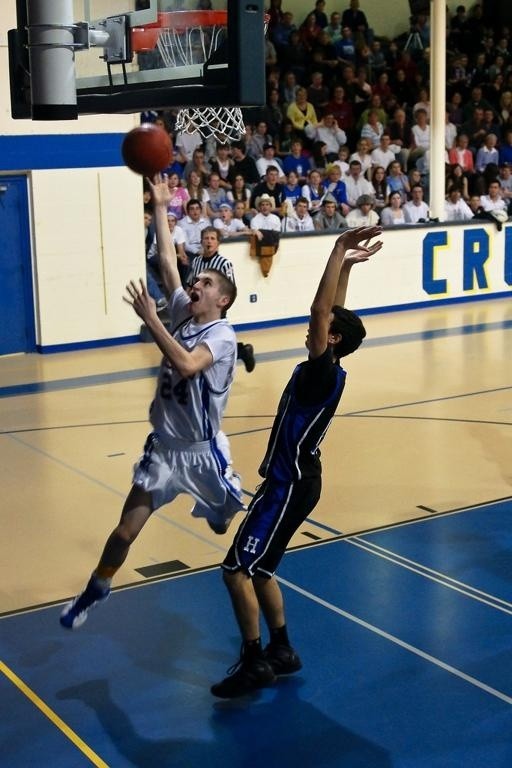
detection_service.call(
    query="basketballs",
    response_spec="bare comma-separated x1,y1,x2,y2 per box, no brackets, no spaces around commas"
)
122,124,173,176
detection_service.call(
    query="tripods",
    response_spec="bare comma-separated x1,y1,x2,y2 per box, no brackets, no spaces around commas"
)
403,27,424,51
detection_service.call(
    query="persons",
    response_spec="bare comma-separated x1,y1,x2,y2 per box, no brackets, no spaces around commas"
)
138,1,512,308
209,219,385,699
183,225,255,372
59,164,246,628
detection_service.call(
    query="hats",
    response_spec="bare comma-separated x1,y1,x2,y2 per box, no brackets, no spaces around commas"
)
255,193,276,211
322,192,337,203
262,141,277,152
356,194,374,206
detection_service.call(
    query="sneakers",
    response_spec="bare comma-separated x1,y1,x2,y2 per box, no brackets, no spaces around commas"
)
262,644,304,676
59,569,112,631
210,657,277,698
241,343,256,373
